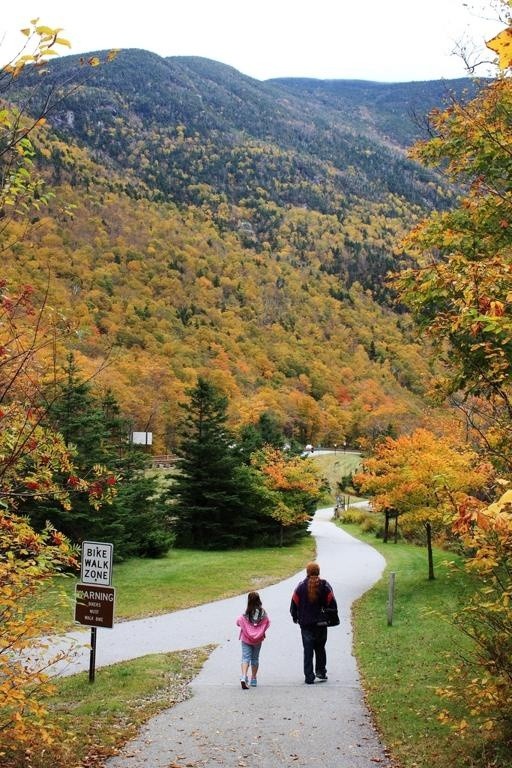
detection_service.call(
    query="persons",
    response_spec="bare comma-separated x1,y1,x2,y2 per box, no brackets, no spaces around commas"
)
289,561,339,685
235,591,271,689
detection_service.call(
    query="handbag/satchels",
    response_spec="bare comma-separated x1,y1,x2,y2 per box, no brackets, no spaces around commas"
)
317,609,339,627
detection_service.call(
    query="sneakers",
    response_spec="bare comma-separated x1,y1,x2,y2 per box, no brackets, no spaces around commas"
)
240,676,256,689
316,673,328,680
